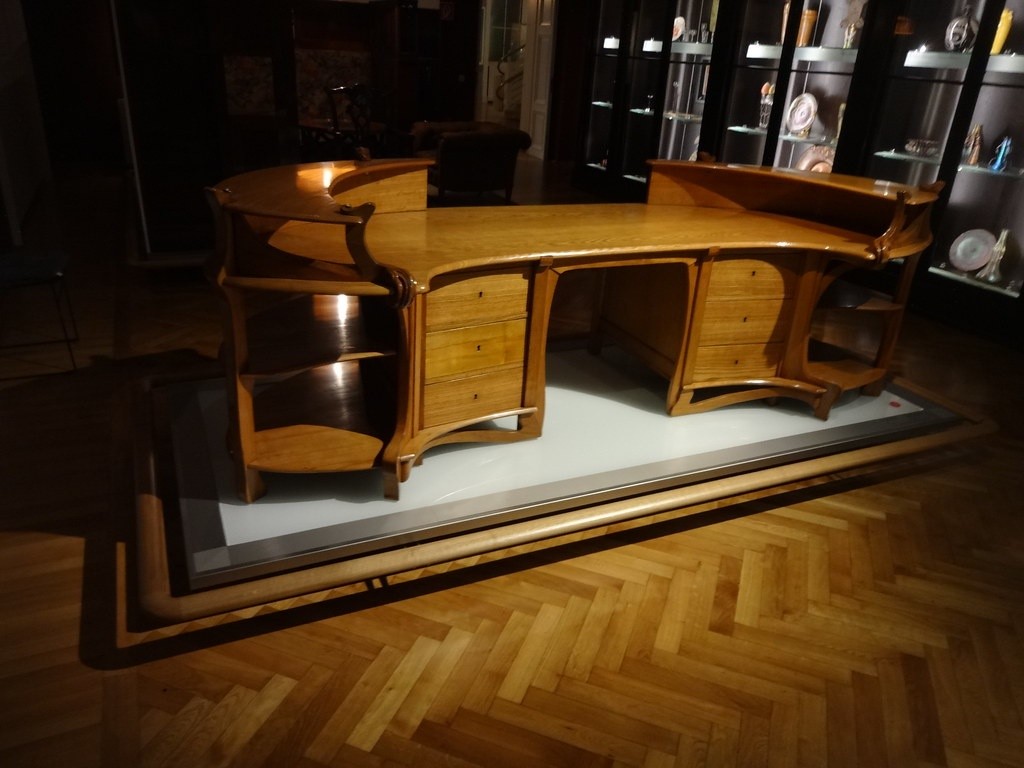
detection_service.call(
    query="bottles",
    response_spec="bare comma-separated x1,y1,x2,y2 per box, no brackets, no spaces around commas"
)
945,5,979,55
976,228,1010,285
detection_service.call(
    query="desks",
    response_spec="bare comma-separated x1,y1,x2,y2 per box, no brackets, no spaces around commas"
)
300,118,385,159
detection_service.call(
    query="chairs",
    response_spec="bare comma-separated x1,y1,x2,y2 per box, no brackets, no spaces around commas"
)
410,121,531,203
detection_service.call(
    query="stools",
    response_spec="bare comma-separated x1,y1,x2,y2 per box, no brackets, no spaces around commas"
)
1,244,81,384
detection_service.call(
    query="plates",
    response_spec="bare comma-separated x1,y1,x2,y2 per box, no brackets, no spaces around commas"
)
786,93,818,133
796,145,836,174
950,230,996,270
672,17,684,40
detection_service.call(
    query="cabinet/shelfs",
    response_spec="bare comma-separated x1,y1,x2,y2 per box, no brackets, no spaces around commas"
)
556,0,1024,303
209,158,435,504
646,159,947,423
591,239,832,422
386,263,557,480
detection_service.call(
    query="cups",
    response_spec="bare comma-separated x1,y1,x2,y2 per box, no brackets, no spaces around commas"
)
990,9,1012,55
796,10,816,47
